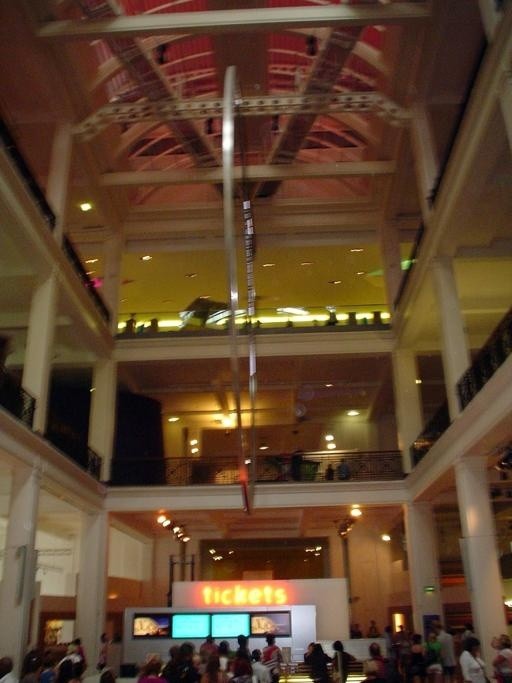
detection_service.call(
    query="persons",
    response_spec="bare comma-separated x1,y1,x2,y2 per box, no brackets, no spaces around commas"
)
116,310,388,337
0,629,285,683
286,445,350,480
304,618,512,683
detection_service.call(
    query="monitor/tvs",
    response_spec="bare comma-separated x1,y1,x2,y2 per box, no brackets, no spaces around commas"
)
132,613,171,639
250,611,292,638
